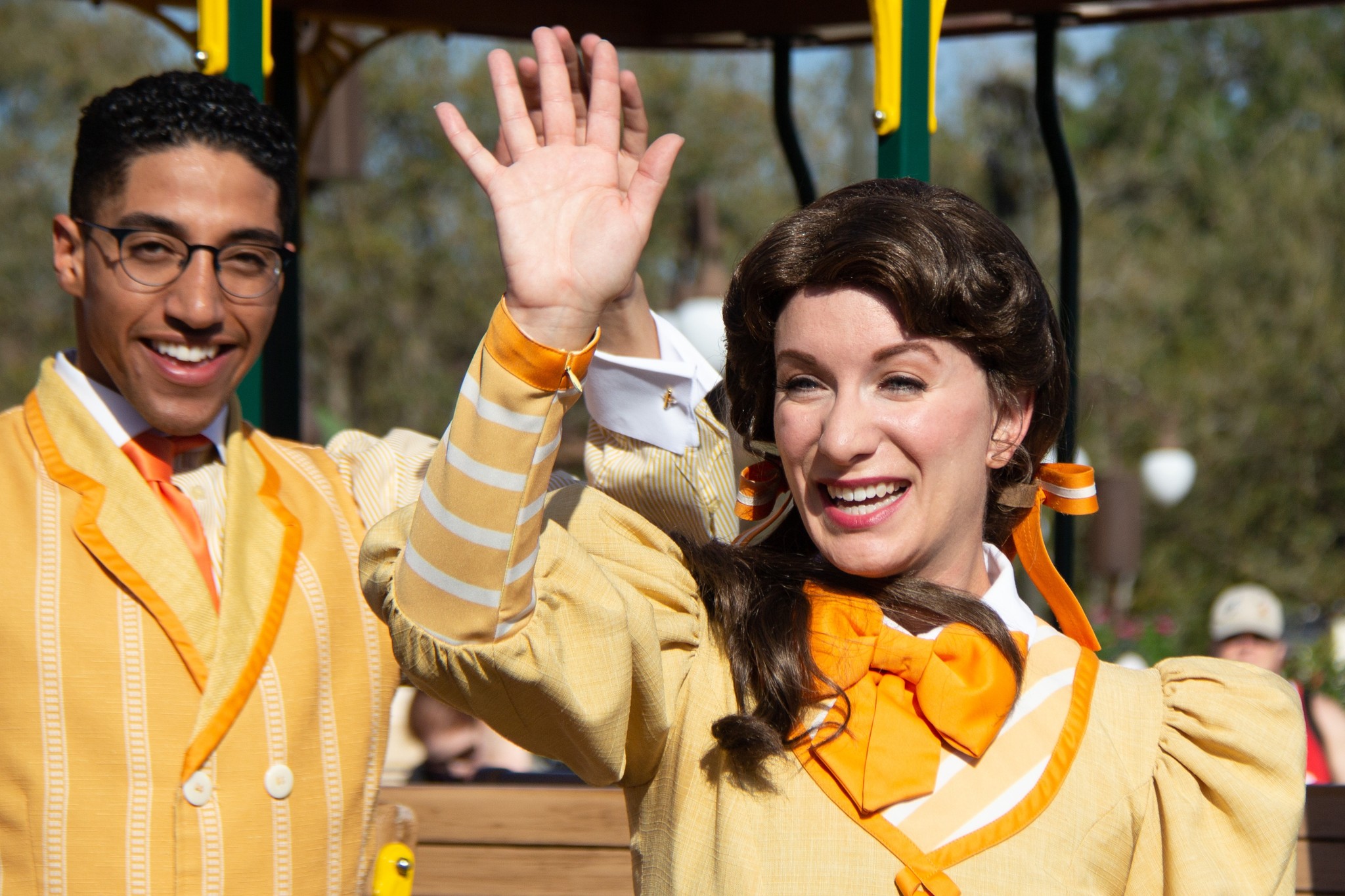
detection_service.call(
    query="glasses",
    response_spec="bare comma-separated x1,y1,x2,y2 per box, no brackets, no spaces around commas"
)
74,215,293,297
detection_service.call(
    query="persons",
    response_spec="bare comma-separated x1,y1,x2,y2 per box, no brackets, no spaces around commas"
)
0,27,767,896
358,27,1308,896
1209,583,1345,786
406,689,494,783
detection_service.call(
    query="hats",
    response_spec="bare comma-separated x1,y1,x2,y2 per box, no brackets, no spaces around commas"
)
1210,587,1284,644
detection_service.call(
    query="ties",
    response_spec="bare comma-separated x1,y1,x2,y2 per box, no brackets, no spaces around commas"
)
121,431,219,613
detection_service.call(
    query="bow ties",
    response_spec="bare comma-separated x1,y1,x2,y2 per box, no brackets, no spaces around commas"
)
794,584,1030,814
